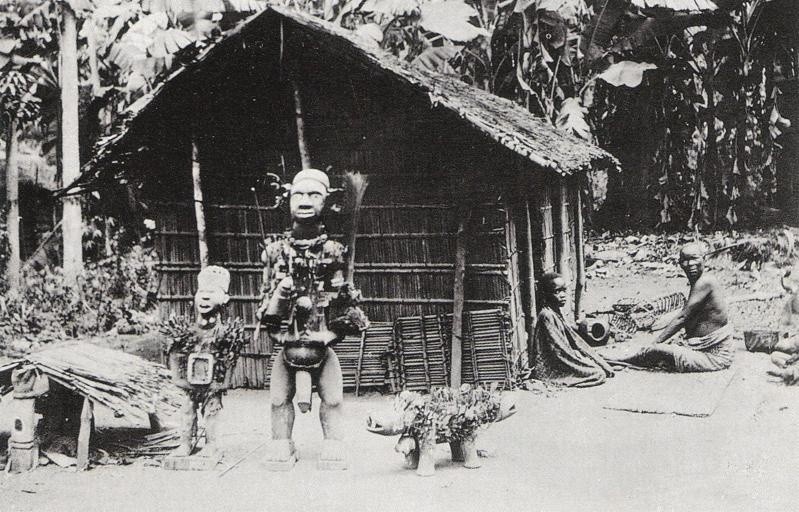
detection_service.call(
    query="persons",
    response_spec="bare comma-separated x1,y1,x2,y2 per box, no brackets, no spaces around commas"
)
166,264,250,455
596,241,735,374
528,268,624,388
254,167,373,475
769,279,799,388
